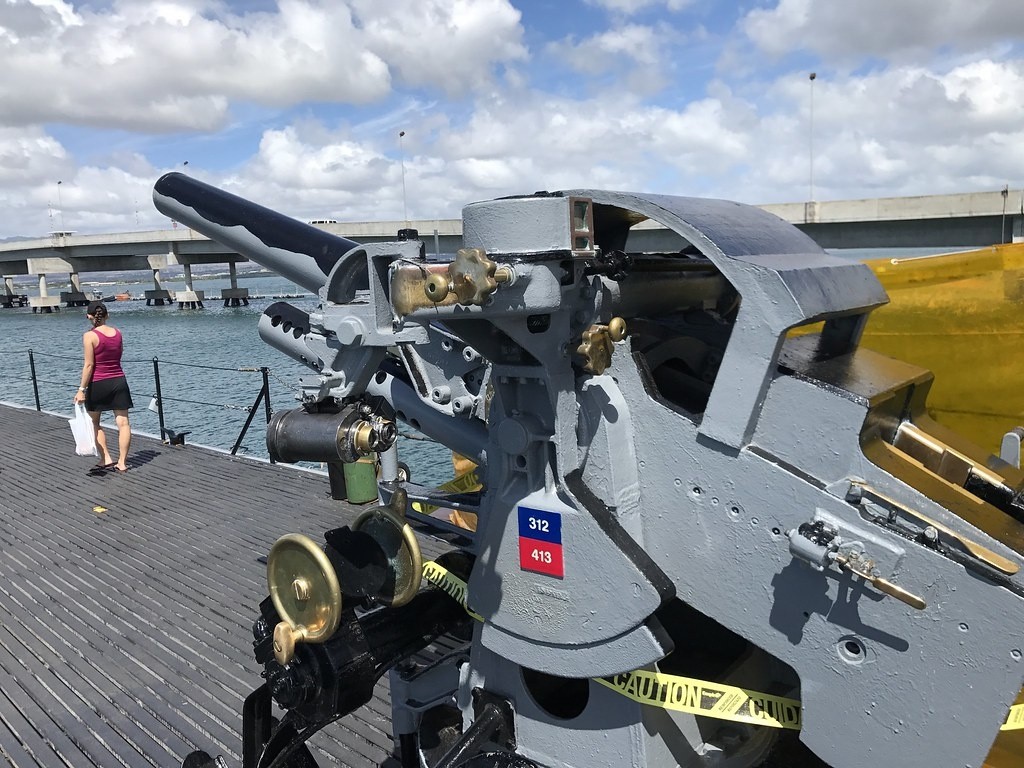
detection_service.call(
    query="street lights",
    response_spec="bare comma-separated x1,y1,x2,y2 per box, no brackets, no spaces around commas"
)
399,131,410,229
810,71,817,201
183,160,193,239
58,181,67,245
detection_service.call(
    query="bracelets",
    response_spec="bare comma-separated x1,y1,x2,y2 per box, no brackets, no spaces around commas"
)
79,387,87,392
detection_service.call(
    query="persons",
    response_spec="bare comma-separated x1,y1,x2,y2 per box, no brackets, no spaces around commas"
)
74,301,134,472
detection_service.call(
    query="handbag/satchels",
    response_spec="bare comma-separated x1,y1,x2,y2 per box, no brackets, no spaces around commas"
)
68,402,98,457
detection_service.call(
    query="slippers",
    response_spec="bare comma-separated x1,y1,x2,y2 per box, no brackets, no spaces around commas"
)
89,463,113,472
113,464,127,472
